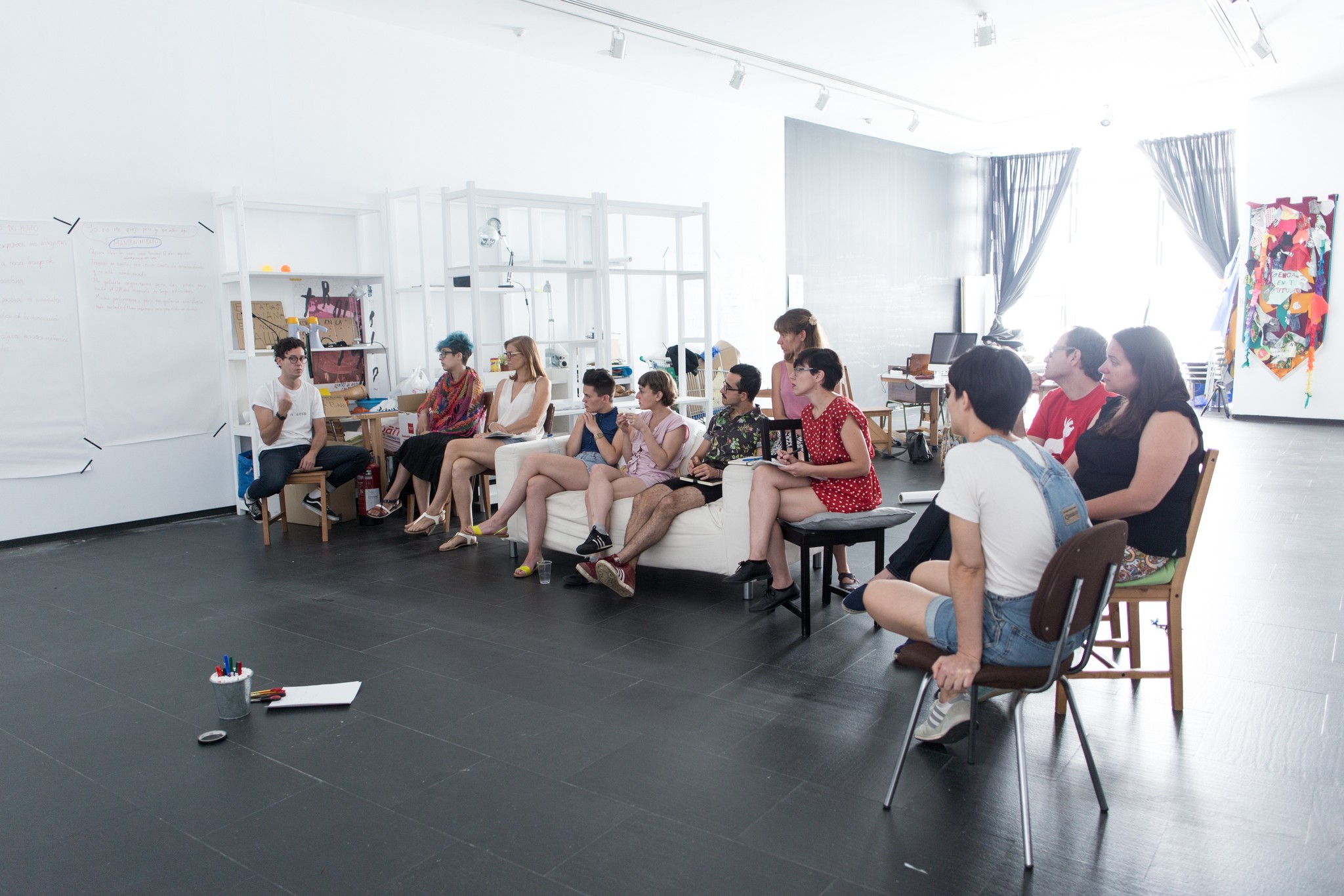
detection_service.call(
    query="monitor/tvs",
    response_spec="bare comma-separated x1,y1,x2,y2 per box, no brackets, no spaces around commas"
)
929,333,978,365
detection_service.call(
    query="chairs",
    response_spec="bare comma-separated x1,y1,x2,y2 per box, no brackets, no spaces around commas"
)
883,518,1129,870
261,468,332,545
1055,448,1220,717
472,404,556,521
843,365,892,456
1183,333,1224,412
404,391,494,532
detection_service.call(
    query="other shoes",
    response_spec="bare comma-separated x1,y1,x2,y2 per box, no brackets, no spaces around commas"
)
894,638,921,659
841,584,867,614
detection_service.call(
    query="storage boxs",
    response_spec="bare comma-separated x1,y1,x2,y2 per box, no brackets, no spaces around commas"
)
398,393,431,448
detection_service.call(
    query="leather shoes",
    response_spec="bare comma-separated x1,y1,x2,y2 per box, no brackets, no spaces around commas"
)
748,582,800,613
722,559,772,584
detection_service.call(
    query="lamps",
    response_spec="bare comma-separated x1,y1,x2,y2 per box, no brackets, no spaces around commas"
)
908,110,920,132
730,62,747,90
815,86,830,110
542,280,555,366
610,25,626,60
477,217,517,287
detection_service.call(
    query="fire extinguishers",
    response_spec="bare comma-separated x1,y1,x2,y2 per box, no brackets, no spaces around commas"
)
355,450,384,526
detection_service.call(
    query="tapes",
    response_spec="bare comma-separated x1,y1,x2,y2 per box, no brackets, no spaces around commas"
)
198,730,227,744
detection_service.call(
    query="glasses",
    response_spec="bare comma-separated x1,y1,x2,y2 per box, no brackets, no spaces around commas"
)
285,356,308,365
439,351,465,358
723,381,740,394
1049,346,1076,357
945,383,958,398
504,351,522,358
794,367,816,376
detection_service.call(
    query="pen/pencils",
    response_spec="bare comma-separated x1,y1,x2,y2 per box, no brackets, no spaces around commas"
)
779,449,801,459
742,457,763,460
216,655,242,677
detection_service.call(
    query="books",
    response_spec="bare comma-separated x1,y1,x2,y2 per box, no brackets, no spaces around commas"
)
728,456,788,470
485,431,514,438
680,474,722,487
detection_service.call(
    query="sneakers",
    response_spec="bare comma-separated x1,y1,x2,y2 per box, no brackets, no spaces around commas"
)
575,553,623,584
303,493,339,521
977,686,1016,704
915,693,970,742
576,525,613,555
244,494,271,524
595,560,636,597
562,571,593,584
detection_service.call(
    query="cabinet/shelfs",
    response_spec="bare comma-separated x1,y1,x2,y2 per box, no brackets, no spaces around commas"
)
437,184,715,425
218,187,397,516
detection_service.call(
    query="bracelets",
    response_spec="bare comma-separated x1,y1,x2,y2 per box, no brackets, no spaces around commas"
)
716,469,720,478
487,421,496,433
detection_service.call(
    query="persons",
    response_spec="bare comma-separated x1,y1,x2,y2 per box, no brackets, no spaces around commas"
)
723,348,881,613
576,364,773,596
366,331,482,535
839,326,1205,747
463,369,621,577
404,336,552,552
244,336,371,525
561,371,689,585
771,308,860,590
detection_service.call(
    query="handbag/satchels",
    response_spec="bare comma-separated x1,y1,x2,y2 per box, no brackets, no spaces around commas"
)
906,432,934,464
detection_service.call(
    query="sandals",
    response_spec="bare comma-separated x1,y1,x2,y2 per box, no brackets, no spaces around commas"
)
514,558,545,577
463,525,509,538
404,510,446,534
367,499,403,517
439,532,478,551
425,525,436,535
837,573,860,591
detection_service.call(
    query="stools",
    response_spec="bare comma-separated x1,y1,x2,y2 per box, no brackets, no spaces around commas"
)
776,524,889,636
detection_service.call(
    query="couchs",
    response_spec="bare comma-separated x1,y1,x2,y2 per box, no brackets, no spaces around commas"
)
494,411,821,600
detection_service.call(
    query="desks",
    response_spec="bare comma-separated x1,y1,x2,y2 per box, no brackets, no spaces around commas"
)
877,373,949,451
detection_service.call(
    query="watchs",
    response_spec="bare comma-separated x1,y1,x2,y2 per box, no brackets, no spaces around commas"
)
594,433,605,442
276,411,288,421
